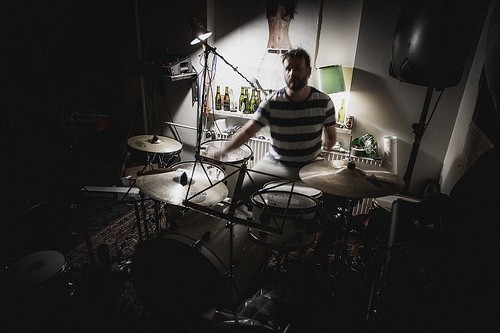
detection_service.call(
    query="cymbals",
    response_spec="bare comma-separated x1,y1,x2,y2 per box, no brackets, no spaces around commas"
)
136,171,229,208
299,158,406,199
127,133,182,154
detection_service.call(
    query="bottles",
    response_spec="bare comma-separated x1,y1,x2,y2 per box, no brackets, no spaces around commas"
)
337,106,345,123
239,86,245,111
256,90,261,110
249,89,256,114
215,86,221,110
244,89,250,114
269,89,272,94
223,86,230,111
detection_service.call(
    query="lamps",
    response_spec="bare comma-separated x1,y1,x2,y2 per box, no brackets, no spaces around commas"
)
188,16,260,94
317,64,347,95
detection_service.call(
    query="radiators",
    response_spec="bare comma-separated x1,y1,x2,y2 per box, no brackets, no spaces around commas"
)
206,132,384,215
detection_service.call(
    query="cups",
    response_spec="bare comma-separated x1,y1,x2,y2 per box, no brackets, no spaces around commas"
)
346,115,354,129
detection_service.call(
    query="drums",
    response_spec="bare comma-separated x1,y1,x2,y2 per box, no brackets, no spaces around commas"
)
167,160,227,184
0,248,67,293
194,137,254,196
262,179,323,203
158,197,272,313
248,190,318,249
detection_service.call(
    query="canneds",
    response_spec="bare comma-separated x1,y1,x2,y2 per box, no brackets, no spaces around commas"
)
346,116,354,128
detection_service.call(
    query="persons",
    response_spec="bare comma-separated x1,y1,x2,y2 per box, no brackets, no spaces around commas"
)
210,48,337,211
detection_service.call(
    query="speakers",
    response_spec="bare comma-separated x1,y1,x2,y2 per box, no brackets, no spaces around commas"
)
389,0,482,92
